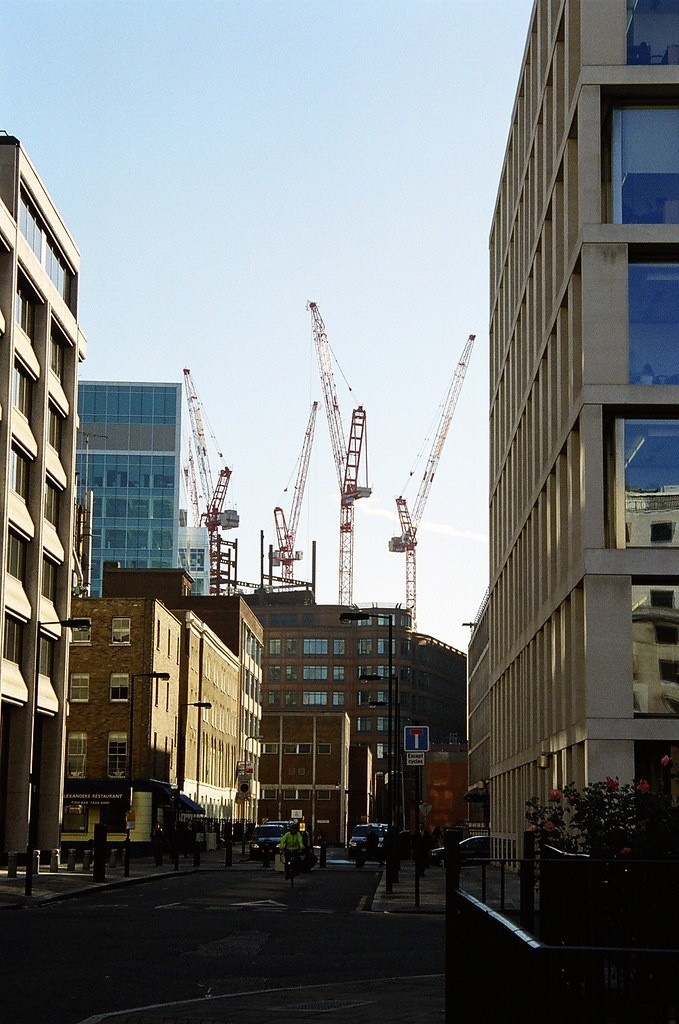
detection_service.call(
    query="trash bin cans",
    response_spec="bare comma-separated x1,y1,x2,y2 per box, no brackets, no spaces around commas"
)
206,833,216,851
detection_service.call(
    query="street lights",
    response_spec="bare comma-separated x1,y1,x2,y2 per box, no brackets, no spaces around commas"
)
124,672,171,858
239,735,264,858
357,674,397,785
340,610,394,828
368,702,403,779
24,618,92,899
171,703,212,864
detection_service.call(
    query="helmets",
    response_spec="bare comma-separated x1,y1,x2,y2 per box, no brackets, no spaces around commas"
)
289,823,297,833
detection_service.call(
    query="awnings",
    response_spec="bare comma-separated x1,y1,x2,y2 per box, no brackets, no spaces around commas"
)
178,796,204,813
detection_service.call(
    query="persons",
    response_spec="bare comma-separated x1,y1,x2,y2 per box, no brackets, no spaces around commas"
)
419,823,430,876
280,824,306,880
367,825,384,867
432,825,443,846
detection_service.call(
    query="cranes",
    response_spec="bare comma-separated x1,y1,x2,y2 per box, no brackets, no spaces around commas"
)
266,401,320,590
305,298,373,606
387,334,476,632
182,366,242,594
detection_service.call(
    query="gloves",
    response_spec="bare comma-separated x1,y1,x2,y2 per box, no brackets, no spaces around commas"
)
283,848,287,851
302,849,306,854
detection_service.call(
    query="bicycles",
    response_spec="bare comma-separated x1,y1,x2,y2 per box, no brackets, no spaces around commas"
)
277,847,301,887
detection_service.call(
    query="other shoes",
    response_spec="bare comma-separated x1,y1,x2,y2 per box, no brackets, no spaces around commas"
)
295,871,299,876
285,874,289,880
378,863,385,867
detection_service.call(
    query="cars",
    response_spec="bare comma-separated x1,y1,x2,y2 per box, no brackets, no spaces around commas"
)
250,825,285,858
431,836,488,867
348,823,393,859
